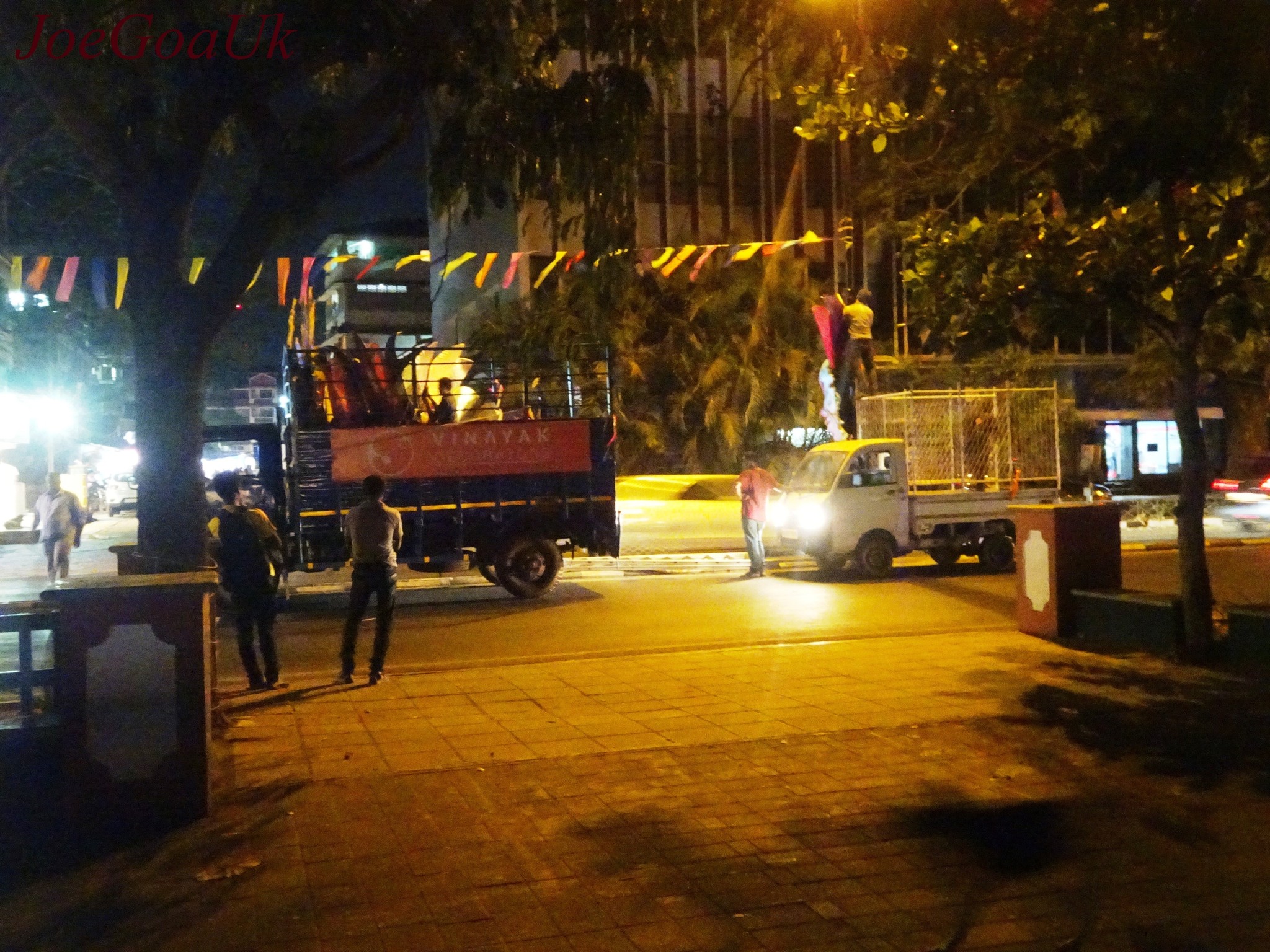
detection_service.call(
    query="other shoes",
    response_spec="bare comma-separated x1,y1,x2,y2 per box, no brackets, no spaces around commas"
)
741,570,765,578
368,671,390,686
339,671,353,683
250,681,266,693
265,679,289,691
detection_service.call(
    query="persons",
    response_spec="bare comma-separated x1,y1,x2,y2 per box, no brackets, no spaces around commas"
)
205,470,292,691
841,288,879,400
735,451,792,579
962,396,1002,494
422,376,459,424
331,475,404,686
30,471,87,582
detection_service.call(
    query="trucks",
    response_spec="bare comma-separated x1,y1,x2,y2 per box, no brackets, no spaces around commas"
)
201,320,621,597
779,380,1064,581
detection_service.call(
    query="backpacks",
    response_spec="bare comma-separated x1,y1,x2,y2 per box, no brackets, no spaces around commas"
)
215,505,270,593
